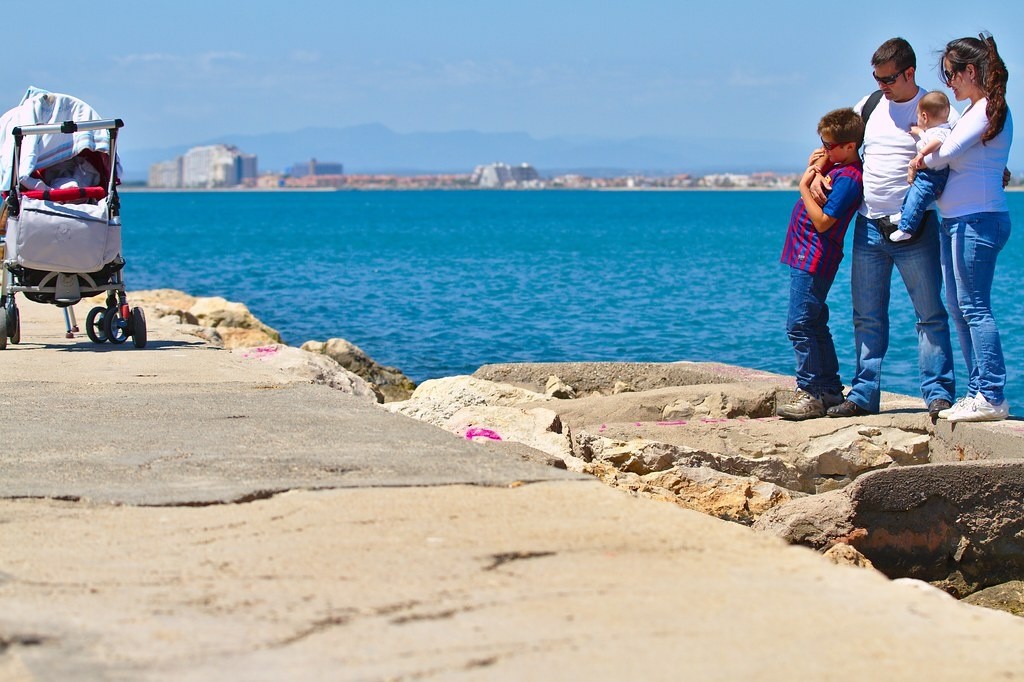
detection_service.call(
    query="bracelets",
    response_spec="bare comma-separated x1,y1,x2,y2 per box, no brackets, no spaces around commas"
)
909,161,913,168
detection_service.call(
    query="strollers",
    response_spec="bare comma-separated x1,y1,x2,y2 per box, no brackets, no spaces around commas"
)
1,118,147,350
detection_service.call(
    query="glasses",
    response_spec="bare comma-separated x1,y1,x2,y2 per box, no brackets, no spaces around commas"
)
821,136,840,150
945,63,979,81
873,69,905,85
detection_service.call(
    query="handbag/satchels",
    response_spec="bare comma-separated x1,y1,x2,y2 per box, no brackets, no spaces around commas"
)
878,210,933,244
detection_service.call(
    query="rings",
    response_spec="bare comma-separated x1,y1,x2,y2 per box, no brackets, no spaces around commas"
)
910,179,913,180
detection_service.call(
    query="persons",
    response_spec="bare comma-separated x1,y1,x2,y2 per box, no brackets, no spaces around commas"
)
809,37,1012,417
777,108,865,420
907,33,1011,423
890,91,951,241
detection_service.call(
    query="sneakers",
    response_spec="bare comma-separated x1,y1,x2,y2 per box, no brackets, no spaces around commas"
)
777,390,827,421
826,401,872,416
928,399,952,417
822,400,844,415
947,392,1008,423
938,397,974,419
